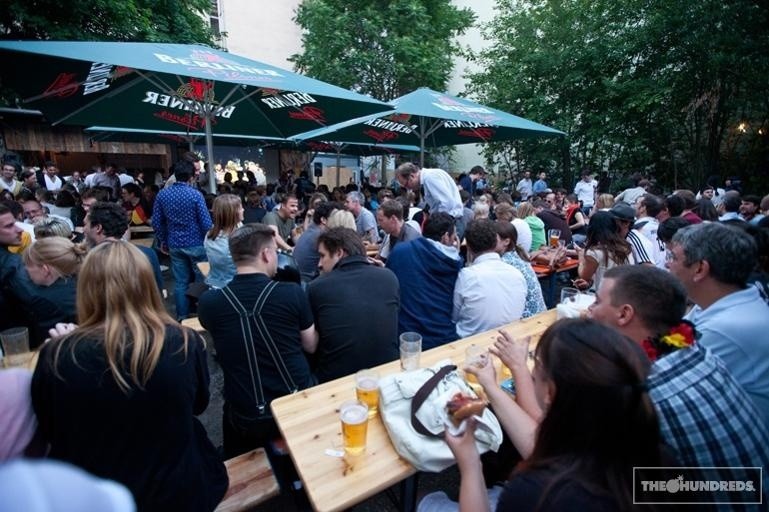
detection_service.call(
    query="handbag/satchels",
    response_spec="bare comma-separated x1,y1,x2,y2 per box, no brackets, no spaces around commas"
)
378,359,504,473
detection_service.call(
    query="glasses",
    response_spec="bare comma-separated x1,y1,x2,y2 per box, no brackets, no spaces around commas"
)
543,198,552,203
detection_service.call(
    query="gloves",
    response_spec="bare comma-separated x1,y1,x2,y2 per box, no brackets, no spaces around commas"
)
271,266,300,285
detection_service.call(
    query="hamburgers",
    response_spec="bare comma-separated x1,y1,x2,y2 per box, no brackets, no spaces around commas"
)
445,393,485,429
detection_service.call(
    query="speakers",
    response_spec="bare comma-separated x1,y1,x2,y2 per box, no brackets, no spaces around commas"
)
314,162,322,176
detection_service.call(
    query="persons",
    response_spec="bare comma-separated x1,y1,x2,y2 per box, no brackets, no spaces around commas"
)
0,152,769,512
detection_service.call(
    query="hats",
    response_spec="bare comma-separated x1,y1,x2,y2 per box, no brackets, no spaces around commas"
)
606,204,637,222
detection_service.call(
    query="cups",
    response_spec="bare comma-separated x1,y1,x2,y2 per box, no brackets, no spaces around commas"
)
339,398,369,457
547,229,561,249
0,326,32,369
500,360,513,380
399,332,422,371
354,368,381,419
464,344,489,385
560,287,583,313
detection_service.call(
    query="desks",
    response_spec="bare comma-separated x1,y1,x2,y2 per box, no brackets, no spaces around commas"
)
532,257,580,308
0,350,40,371
196,249,379,279
270,307,558,512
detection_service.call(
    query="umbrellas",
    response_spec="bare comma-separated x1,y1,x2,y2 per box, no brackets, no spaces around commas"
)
0,41,397,193
291,85,568,170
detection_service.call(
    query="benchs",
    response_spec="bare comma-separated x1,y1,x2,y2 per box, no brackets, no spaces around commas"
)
181,317,206,333
216,447,280,511
